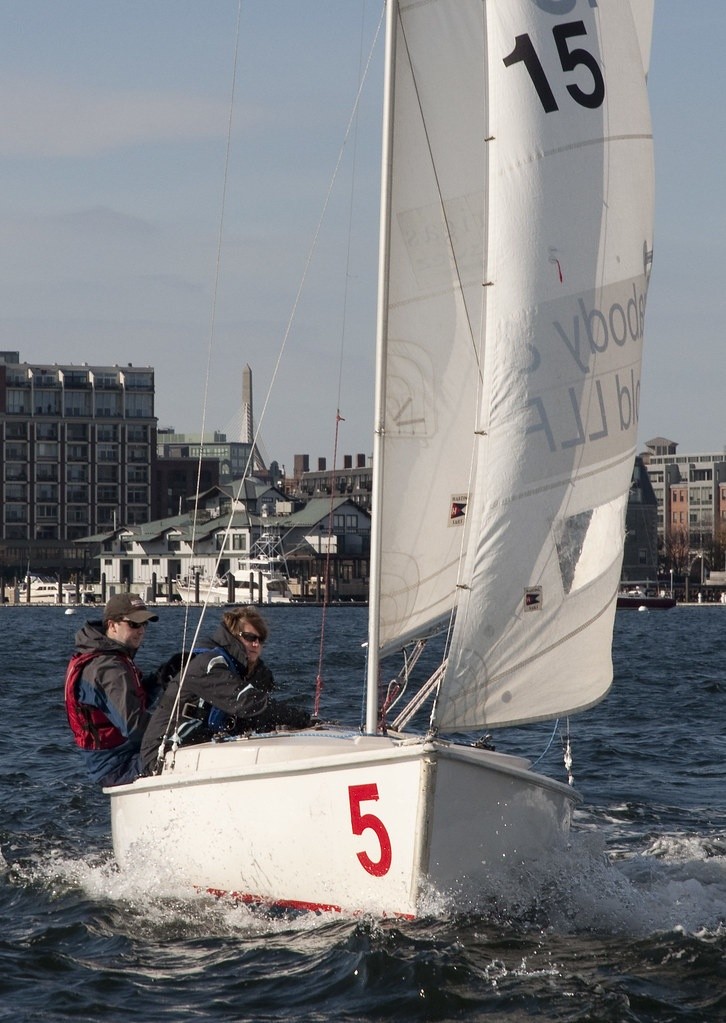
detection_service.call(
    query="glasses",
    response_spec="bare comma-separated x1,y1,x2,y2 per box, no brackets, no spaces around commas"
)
115,620,148,629
239,632,266,644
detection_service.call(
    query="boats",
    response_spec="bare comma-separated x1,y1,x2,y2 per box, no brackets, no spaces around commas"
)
4,560,95,603
176,523,299,604
617,586,676,608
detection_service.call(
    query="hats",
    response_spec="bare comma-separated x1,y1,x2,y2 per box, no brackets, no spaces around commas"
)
103,592,159,623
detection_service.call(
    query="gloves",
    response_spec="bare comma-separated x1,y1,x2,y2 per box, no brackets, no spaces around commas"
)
295,711,311,729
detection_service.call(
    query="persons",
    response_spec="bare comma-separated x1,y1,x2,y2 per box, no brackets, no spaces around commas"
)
139,607,322,773
64,592,195,786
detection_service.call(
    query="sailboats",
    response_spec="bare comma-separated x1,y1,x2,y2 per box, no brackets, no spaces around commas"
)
101,0,656,921
